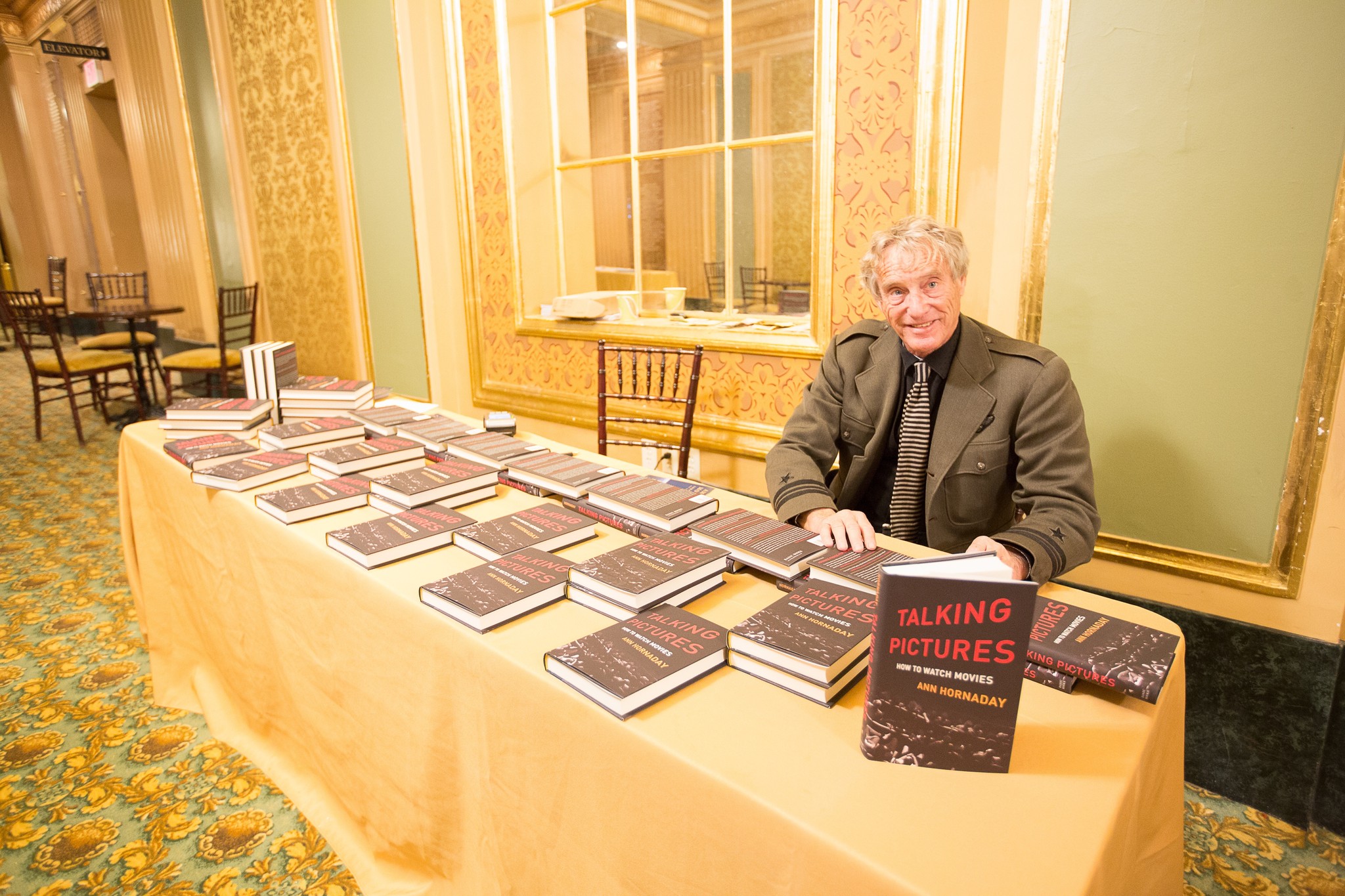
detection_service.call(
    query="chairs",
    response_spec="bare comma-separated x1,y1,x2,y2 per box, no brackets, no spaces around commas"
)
705,259,781,316
595,337,702,479
85,271,168,410
0,285,149,444
160,280,258,406
8,257,78,349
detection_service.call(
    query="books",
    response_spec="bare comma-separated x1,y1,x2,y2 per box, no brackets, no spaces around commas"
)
419,547,582,634
451,503,600,563
190,448,311,493
164,433,260,472
254,472,378,525
858,551,1039,774
543,603,729,720
160,340,1182,709
325,503,476,571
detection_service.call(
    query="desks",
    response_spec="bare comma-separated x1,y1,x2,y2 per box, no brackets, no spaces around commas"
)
762,278,810,291
117,395,1190,896
68,301,185,430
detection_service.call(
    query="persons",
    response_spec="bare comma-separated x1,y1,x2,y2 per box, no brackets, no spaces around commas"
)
765,218,1102,587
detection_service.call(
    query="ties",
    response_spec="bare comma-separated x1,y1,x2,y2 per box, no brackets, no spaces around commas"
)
889,361,931,545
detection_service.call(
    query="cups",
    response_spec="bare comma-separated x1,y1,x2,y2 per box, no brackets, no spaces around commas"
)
615,291,640,322
663,287,687,317
